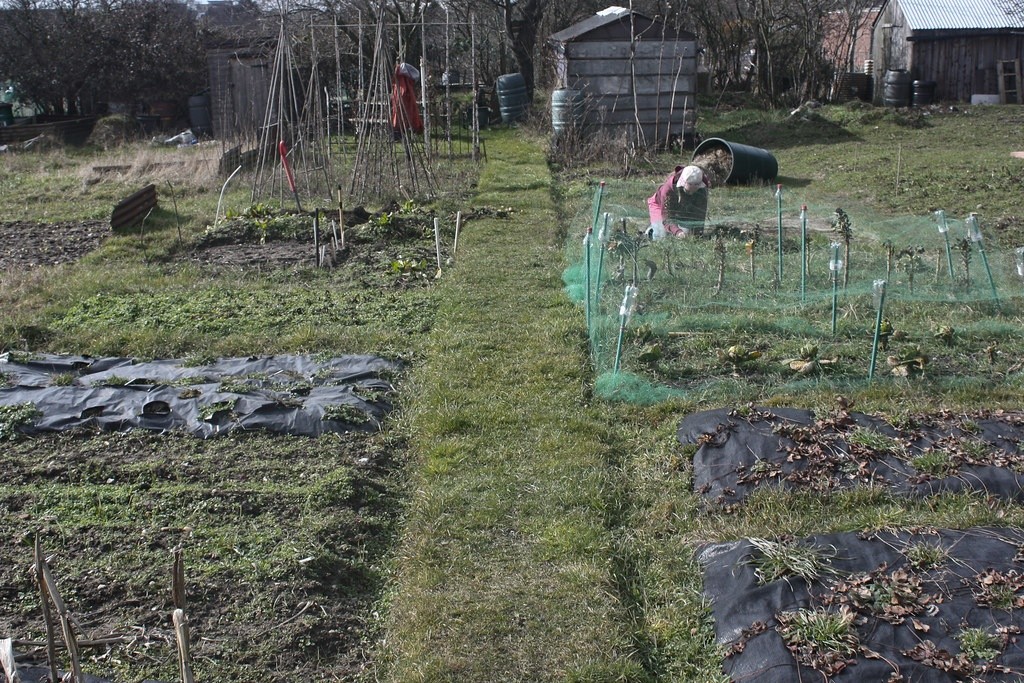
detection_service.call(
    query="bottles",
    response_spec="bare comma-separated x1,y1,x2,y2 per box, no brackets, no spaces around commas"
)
583,228,594,263
829,242,843,282
799,205,809,225
1016,246,1024,277
597,212,613,251
773,184,784,201
934,210,948,242
965,217,985,253
619,286,643,330
597,180,608,197
872,279,886,311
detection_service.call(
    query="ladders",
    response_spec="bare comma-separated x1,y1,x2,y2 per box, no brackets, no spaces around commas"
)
997,56,1023,104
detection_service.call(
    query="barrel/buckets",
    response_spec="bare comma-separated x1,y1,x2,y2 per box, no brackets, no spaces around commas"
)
691,137,778,187
188,96,213,137
496,72,532,124
883,69,930,108
551,88,584,132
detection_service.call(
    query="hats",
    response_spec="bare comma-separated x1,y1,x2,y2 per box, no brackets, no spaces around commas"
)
676,165,707,190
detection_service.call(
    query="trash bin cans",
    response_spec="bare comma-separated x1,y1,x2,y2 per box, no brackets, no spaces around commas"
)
0,101,14,126
691,138,778,186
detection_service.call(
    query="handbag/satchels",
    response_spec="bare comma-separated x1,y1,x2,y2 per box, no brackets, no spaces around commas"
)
399,62,420,79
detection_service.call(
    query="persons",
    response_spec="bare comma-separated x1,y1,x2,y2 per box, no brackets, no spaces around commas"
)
647,166,707,239
